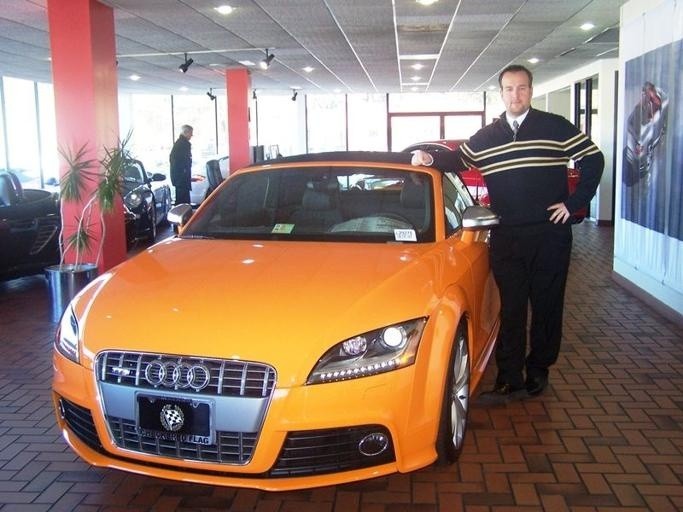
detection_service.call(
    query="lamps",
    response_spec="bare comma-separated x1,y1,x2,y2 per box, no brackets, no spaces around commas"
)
175,49,301,106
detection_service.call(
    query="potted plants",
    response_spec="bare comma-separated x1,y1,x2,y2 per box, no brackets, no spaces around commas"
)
44,125,138,324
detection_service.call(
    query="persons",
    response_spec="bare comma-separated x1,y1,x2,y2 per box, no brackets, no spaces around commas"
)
641,81,661,117
409,66,606,404
169,124,194,234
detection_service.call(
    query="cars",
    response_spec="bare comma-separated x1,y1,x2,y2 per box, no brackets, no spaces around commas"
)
350,139,587,224
51,149,501,495
0,171,62,283
168,156,229,210
623,81,668,179
45,158,172,247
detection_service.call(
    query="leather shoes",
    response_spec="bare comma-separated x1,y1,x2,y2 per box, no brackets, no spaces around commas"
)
477,383,529,404
524,369,549,397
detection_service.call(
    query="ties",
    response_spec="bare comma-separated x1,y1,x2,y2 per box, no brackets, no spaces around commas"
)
512,120,519,140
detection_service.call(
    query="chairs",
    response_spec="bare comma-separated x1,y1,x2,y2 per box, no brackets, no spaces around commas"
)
285,180,427,228
